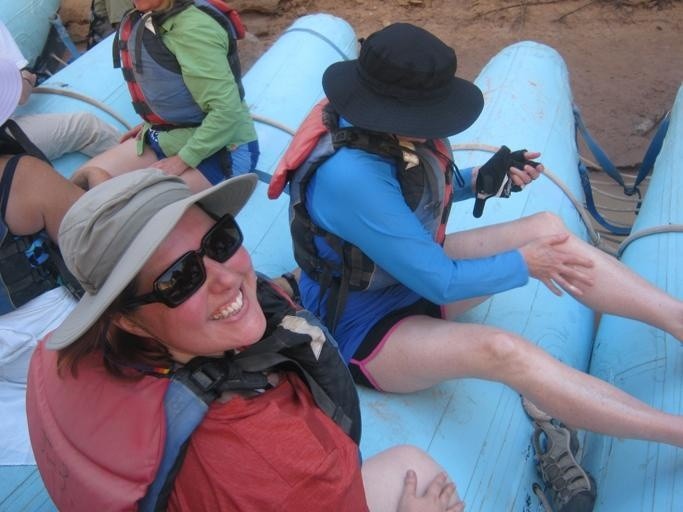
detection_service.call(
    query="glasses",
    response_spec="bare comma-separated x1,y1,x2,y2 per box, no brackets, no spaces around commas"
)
110,209,246,310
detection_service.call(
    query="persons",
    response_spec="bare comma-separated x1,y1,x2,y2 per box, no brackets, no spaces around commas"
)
70,1,260,215
26,164,469,512
267,20,683,446
0,24,128,256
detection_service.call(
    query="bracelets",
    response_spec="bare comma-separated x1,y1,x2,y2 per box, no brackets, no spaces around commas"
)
22,76,33,88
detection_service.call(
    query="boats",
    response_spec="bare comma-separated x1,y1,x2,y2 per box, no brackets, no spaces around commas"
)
12,30,145,179
0,0,60,72
566,43,680,507
0,9,363,512
352,41,594,512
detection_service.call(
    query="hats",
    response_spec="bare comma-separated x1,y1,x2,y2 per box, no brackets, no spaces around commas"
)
320,21,485,141
40,163,262,354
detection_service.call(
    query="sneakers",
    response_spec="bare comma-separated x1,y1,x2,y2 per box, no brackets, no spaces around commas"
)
529,419,598,511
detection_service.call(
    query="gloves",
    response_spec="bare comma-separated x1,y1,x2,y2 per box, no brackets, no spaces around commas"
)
474,143,540,197
